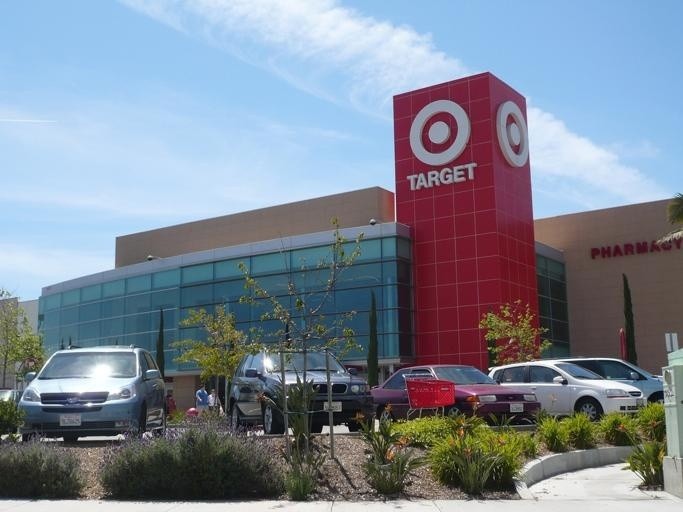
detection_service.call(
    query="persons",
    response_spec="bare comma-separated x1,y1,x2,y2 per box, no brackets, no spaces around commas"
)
166,394,176,414
207,389,220,410
196,383,208,409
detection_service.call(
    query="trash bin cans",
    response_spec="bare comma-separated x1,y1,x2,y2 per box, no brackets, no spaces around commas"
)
187,408,198,417
